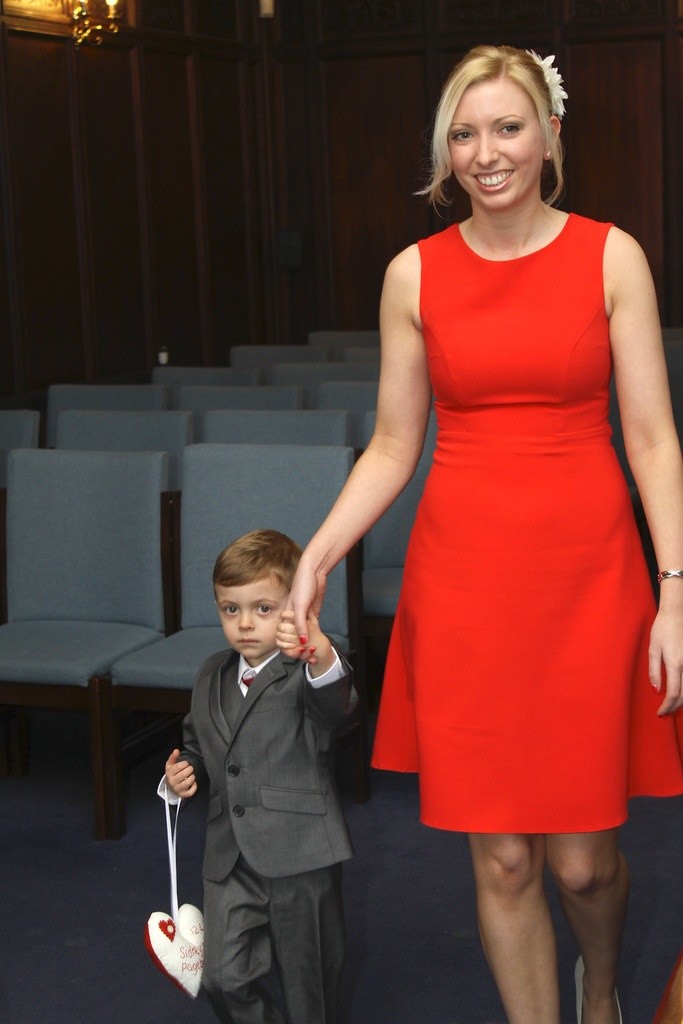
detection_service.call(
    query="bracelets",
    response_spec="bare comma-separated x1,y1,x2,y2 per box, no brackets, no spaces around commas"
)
657,569,683,583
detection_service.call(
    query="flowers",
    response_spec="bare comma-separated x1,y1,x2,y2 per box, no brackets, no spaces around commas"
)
523,47,571,123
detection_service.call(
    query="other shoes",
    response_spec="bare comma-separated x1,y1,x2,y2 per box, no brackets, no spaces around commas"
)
574,956,623,1024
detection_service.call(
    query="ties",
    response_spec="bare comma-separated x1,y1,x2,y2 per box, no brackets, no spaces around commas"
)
242,670,257,688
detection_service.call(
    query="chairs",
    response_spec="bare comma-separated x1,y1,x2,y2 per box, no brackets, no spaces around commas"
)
272,362,370,408
177,384,306,444
43,383,168,450
0,408,41,491
0,450,172,842
360,410,437,640
153,365,262,411
53,410,196,491
308,331,379,362
345,346,380,369
203,408,356,445
230,344,334,383
316,380,379,449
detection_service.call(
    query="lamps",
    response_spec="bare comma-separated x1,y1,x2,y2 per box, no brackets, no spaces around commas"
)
61,0,124,51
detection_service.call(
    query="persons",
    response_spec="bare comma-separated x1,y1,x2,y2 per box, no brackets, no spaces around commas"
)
280,44,683,1024
156,529,360,1024
